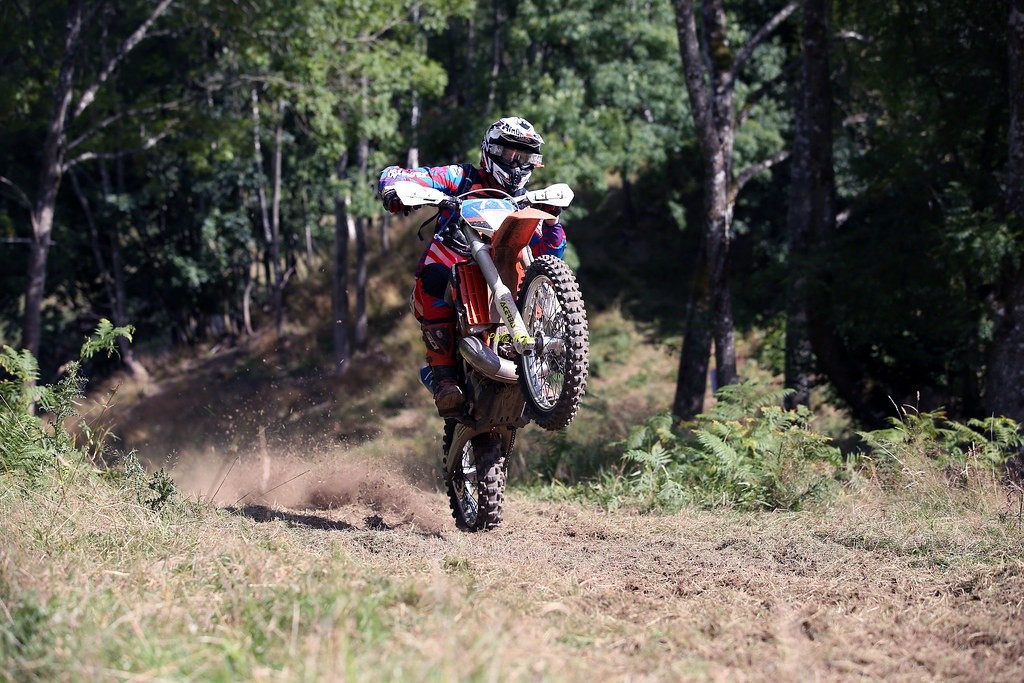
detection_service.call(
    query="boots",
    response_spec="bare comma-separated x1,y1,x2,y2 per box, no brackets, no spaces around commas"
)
422,322,465,409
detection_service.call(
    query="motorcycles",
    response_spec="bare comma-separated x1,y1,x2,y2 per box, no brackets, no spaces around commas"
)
384,180,593,534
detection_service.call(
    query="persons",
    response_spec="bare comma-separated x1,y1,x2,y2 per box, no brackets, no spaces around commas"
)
378,116,568,417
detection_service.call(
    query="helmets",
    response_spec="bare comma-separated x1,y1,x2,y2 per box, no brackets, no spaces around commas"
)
480,116,546,194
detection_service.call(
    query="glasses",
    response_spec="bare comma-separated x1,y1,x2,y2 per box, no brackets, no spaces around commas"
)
480,140,542,169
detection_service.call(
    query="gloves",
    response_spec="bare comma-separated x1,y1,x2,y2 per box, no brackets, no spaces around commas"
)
532,201,570,217
382,192,410,217
